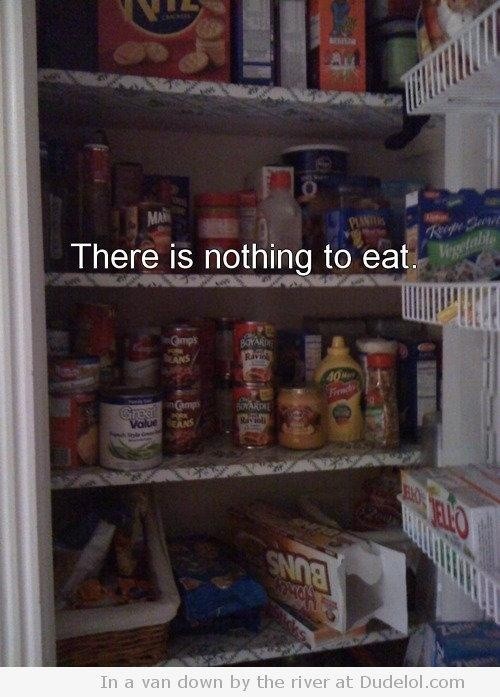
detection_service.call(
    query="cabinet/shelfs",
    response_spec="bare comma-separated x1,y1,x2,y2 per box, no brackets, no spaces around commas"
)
397,0,499,667
0,0,432,666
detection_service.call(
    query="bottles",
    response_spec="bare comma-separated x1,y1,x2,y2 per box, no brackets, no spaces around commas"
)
256,164,306,272
316,335,365,445
365,353,400,447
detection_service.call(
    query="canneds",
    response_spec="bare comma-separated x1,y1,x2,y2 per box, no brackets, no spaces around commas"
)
385,29,419,93
48,142,325,473
